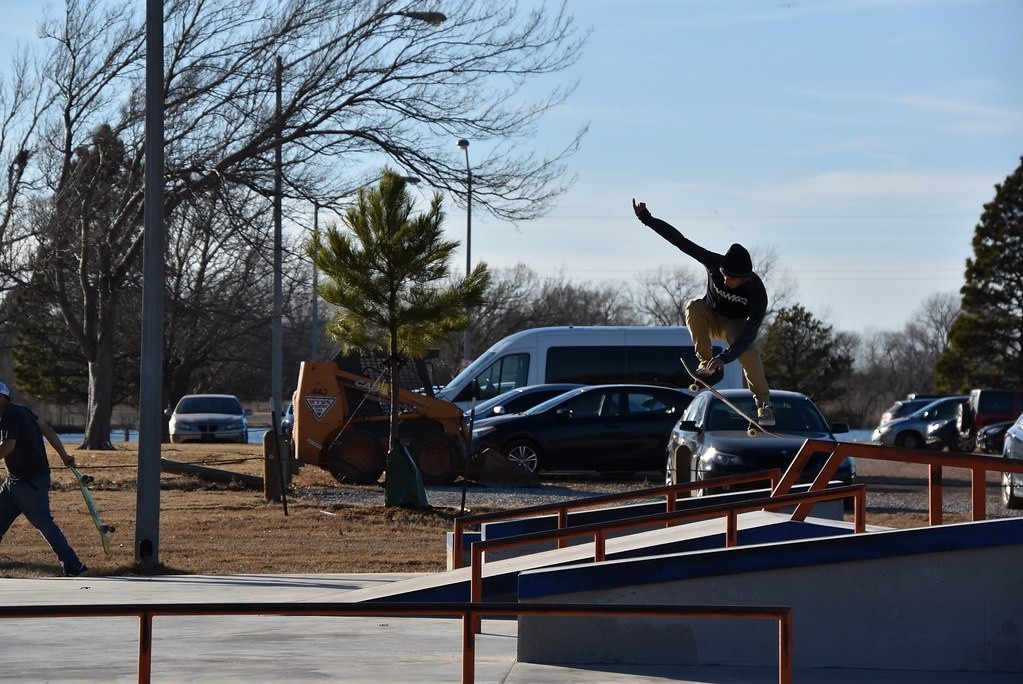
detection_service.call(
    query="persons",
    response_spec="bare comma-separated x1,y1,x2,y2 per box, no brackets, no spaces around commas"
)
0,382,89,580
631,197,778,427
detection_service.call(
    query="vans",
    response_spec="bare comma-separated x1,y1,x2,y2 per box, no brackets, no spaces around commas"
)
433,324,742,415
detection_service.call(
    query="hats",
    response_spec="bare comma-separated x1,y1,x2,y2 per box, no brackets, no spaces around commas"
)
718,243,752,277
0,381,9,398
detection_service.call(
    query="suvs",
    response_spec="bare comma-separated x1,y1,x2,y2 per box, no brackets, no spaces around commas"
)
955,388,1023,438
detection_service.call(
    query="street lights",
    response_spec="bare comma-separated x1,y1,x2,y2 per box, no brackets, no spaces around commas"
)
456,138,473,363
305,173,422,360
265,11,448,489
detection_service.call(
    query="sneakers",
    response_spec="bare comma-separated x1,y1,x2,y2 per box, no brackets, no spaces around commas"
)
756,405,776,425
66,565,89,577
695,361,714,377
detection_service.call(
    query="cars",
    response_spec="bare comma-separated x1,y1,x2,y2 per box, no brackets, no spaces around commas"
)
456,384,591,426
666,389,857,504
976,419,1016,453
459,384,698,481
872,394,970,450
879,397,939,426
164,393,254,445
279,402,295,431
925,416,975,452
1000,414,1022,508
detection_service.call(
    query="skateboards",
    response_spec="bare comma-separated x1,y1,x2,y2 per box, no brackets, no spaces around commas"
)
67,463,115,553
679,356,783,441
